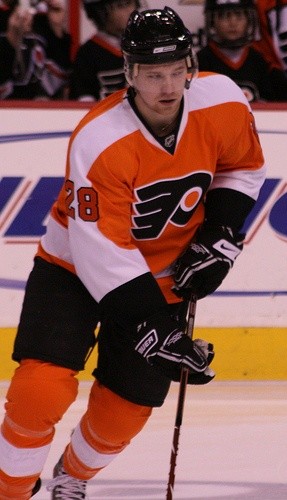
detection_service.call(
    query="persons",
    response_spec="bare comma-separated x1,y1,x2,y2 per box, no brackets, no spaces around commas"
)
251,0,287,73
69,0,140,101
195,0,274,102
0,0,72,100
0,6,268,500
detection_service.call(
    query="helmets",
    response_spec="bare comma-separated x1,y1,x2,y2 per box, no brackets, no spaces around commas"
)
203,0,256,50
85,0,135,30
121,5,194,64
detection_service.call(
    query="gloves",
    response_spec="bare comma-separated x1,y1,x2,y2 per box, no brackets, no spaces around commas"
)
130,314,215,385
170,219,246,302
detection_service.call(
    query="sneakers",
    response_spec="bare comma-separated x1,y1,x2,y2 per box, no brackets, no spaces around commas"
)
29,477,41,500
46,454,87,500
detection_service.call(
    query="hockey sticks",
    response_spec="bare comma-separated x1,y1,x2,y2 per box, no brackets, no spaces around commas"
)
165,293,200,500
